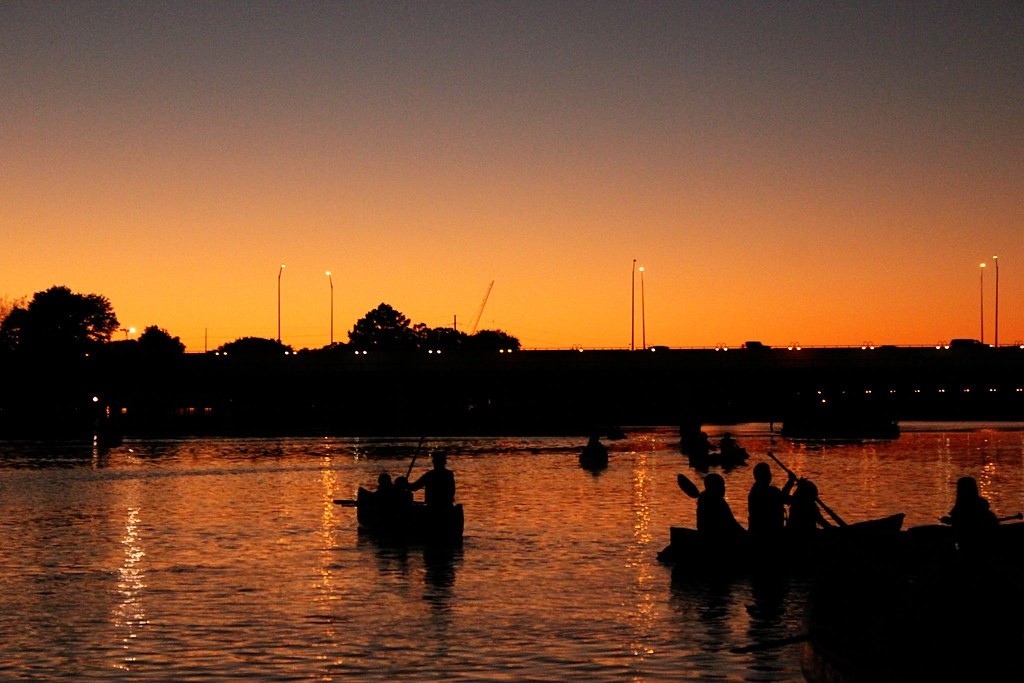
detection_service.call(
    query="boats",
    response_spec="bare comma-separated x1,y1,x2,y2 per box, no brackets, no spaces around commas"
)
798,525,1024,683
668,514,903,569
580,450,609,470
353,488,465,544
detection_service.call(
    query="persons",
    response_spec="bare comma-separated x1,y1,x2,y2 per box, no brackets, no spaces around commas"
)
940,477,989,527
697,462,832,530
701,432,739,454
373,450,455,506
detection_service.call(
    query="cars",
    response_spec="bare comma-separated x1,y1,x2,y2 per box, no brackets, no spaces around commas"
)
744,341,770,349
951,339,983,346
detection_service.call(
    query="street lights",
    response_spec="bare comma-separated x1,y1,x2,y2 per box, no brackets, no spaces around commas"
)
326,271,335,344
277,266,283,343
992,255,999,347
980,263,985,345
638,265,645,351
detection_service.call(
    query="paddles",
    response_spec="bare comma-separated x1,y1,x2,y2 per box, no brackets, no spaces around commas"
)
333,498,361,508
723,627,837,655
766,450,852,528
987,512,1024,524
405,419,436,479
673,472,698,496
